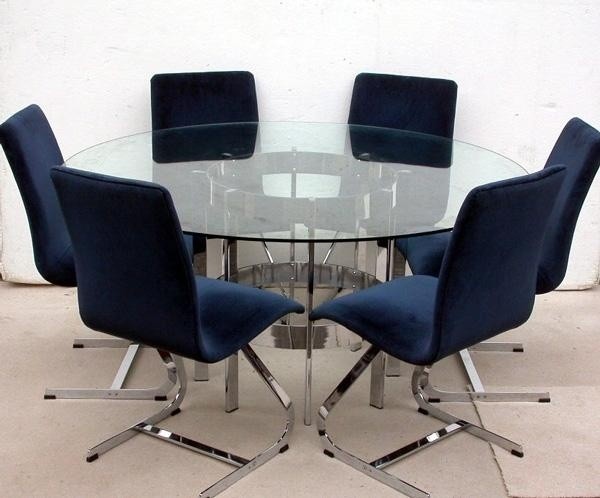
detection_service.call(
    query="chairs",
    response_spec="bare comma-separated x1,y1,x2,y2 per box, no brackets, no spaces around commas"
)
149,71,273,286
305,163,572,498
47,162,296,498
389,116,598,404
0,101,176,405
319,71,459,281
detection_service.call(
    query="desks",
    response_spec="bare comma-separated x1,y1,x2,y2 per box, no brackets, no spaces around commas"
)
62,118,533,422
